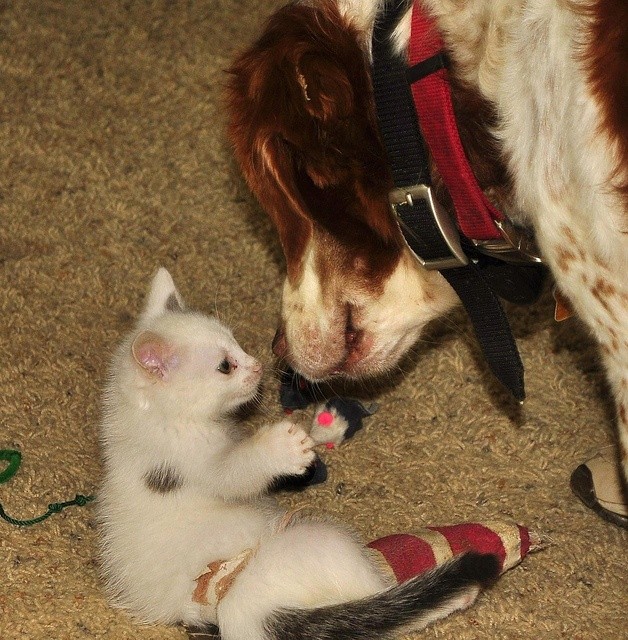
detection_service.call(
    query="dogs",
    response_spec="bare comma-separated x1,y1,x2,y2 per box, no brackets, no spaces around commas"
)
218,0,628,499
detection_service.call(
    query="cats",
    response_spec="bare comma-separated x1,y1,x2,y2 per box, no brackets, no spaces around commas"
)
92,266,502,638
